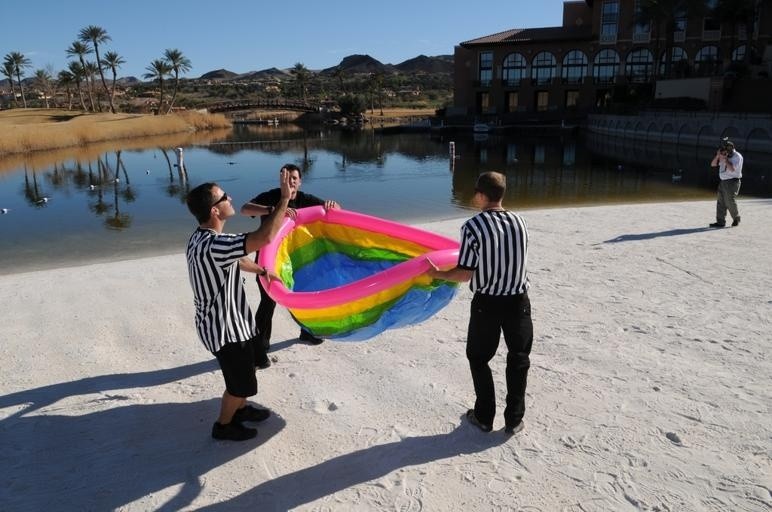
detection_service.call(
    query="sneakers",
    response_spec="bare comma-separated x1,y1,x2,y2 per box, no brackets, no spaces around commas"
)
212,420,257,440
505,420,524,435
257,354,270,369
233,406,269,422
711,220,740,226
299,332,323,344
466,408,493,431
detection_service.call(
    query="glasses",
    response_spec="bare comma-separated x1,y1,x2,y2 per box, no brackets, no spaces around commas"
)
212,192,227,206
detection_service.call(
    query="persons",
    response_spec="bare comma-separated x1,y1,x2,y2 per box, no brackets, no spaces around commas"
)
240,164,342,352
185,168,296,441
710,141,744,227
425,172,533,435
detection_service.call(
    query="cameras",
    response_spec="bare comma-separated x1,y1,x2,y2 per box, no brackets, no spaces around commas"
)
720,137,733,153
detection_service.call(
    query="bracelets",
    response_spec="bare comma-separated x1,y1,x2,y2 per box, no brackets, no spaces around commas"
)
260,266,268,276
268,205,276,214
